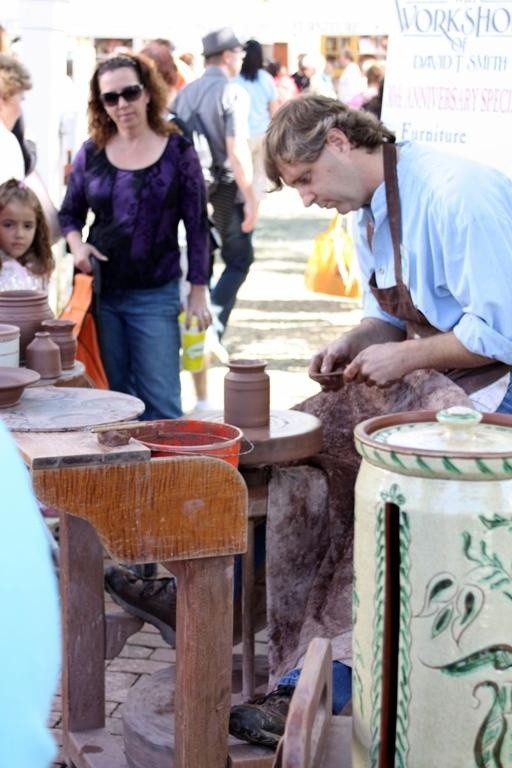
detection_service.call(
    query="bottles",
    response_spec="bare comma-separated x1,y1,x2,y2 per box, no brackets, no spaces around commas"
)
28,332,64,379
222,358,271,430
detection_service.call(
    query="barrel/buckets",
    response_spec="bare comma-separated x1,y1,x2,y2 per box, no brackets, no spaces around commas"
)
100,418,244,476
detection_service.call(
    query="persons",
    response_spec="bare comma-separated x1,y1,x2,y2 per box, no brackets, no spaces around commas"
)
103,95,512,750
0,422,63,768
1,25,385,421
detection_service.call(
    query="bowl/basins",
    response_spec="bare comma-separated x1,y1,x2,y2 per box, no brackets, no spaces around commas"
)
0,367,40,410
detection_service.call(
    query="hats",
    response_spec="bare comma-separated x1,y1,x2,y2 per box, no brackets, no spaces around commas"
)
200,26,250,59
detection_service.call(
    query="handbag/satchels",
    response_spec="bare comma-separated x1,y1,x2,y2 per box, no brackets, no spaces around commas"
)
304,213,361,299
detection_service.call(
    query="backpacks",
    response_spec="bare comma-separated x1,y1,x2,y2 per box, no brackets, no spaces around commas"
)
182,77,225,206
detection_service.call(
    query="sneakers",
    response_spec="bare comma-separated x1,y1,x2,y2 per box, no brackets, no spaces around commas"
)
226,682,334,754
102,561,210,655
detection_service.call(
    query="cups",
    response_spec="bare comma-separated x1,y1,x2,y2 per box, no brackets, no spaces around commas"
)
41,317,78,370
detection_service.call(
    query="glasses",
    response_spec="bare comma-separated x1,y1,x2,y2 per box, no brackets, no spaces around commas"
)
97,84,150,107
